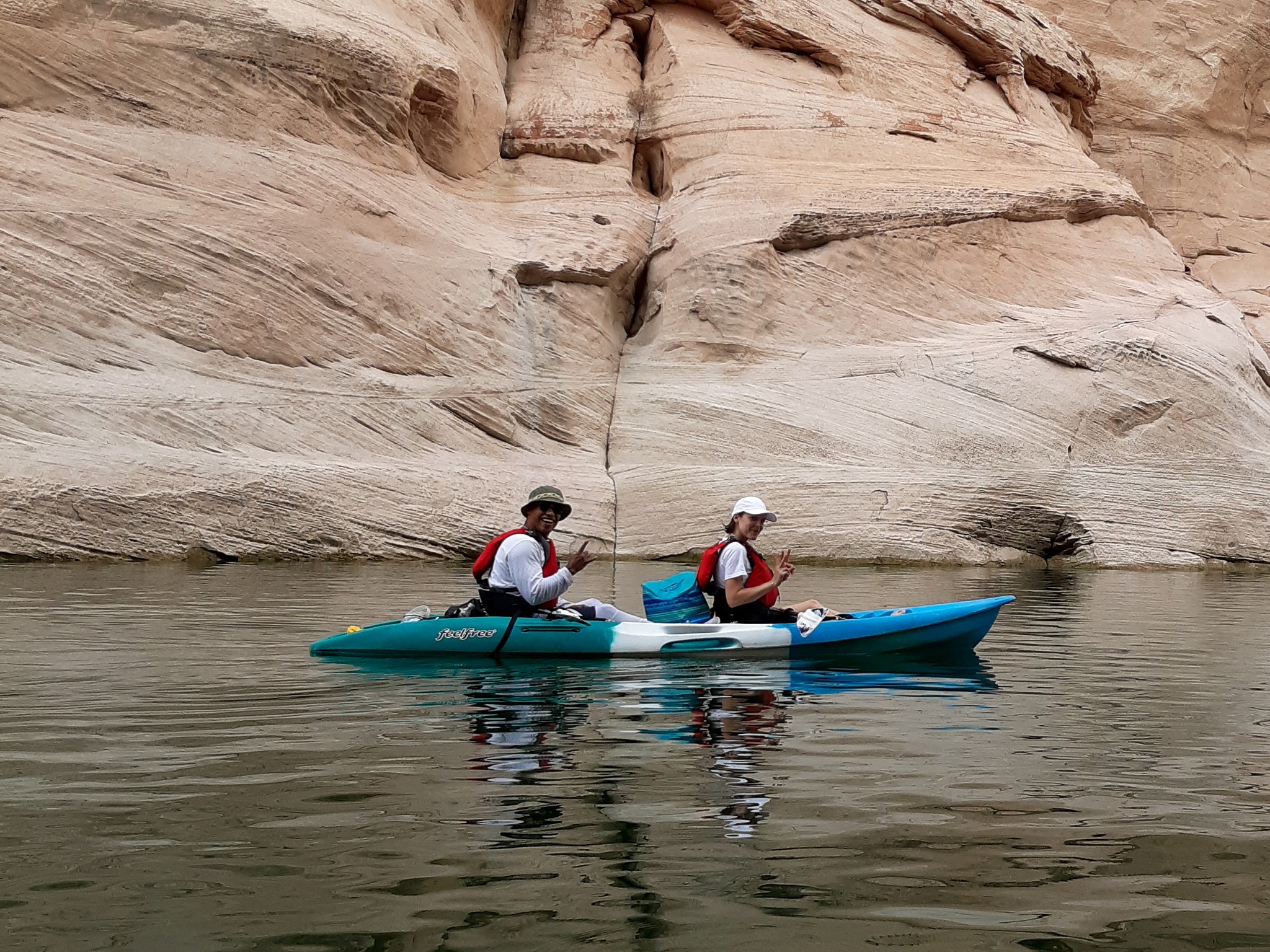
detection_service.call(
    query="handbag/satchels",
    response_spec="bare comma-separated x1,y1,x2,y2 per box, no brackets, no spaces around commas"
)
640,571,711,624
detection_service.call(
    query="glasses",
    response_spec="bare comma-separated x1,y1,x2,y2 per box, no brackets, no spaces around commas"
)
536,504,563,514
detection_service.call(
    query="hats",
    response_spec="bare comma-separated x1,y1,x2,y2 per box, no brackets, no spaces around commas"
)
520,486,572,521
730,497,777,523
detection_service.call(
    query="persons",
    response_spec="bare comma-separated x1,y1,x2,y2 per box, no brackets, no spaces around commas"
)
488,486,720,624
713,497,906,623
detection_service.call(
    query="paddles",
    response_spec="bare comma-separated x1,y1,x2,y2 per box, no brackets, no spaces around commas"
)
535,609,592,626
769,606,830,638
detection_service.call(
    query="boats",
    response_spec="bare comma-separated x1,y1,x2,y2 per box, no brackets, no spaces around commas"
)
308,592,1016,662
319,659,999,696
